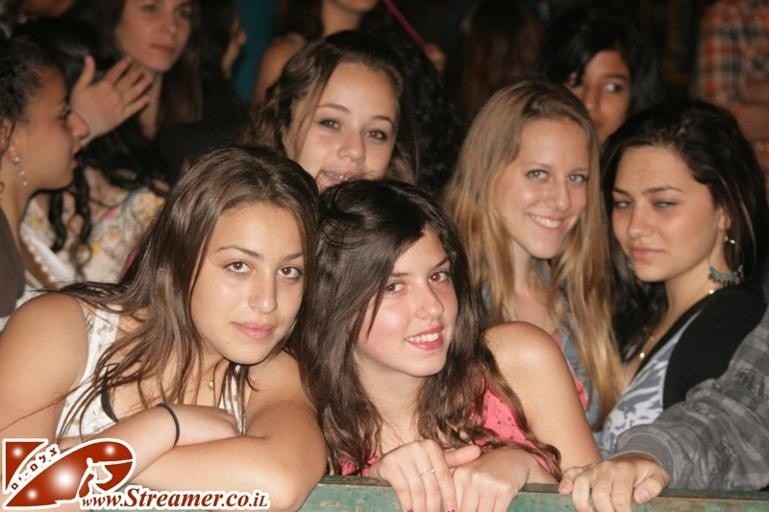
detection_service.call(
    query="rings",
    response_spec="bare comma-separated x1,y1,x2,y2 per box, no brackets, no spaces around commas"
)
421,468,436,478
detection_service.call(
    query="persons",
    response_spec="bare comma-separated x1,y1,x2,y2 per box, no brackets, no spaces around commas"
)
685,1,768,210
1,1,442,328
539,13,667,137
449,76,619,437
556,299,769,512
593,95,750,453
1,144,326,510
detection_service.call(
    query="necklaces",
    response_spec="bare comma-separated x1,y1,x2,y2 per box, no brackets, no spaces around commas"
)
640,324,663,361
295,179,604,512
524,298,549,327
154,359,222,392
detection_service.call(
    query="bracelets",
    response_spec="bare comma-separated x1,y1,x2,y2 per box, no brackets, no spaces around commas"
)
153,402,181,450
